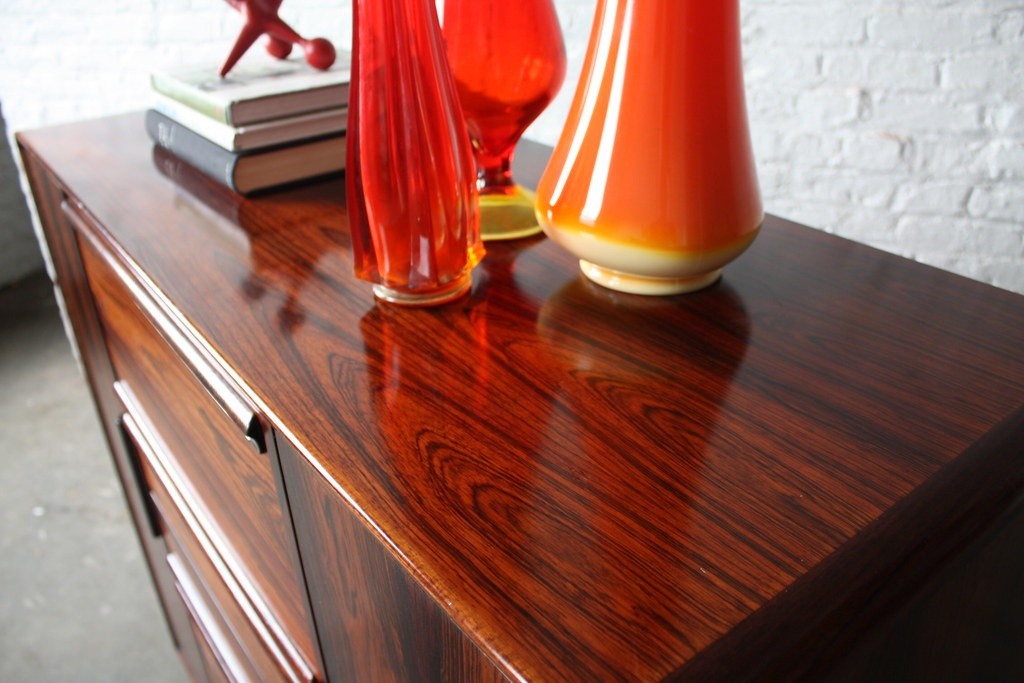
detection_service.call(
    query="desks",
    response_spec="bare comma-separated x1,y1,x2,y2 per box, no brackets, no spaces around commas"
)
15,108,1024,683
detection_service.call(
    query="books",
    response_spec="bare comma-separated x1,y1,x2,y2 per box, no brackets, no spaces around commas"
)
145,58,350,194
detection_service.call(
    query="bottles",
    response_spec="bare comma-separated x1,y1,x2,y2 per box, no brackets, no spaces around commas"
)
534,0,766,296
443,0,567,242
347,0,487,306
